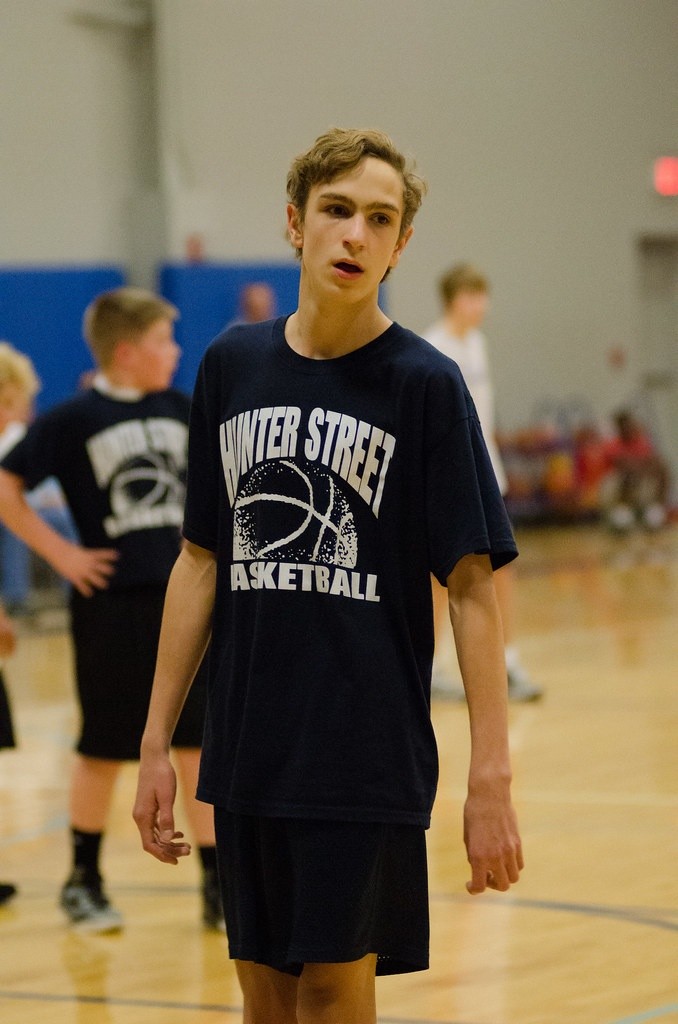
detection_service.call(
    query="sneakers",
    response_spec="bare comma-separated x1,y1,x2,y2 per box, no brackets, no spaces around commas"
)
61,870,121,933
200,893,228,935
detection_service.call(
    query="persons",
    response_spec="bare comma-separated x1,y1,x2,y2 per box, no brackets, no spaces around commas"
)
0,344,42,905
133,125,524,1024
0,286,227,936
421,265,542,703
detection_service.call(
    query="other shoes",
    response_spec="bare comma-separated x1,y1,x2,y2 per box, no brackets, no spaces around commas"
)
500,645,542,702
431,661,470,700
